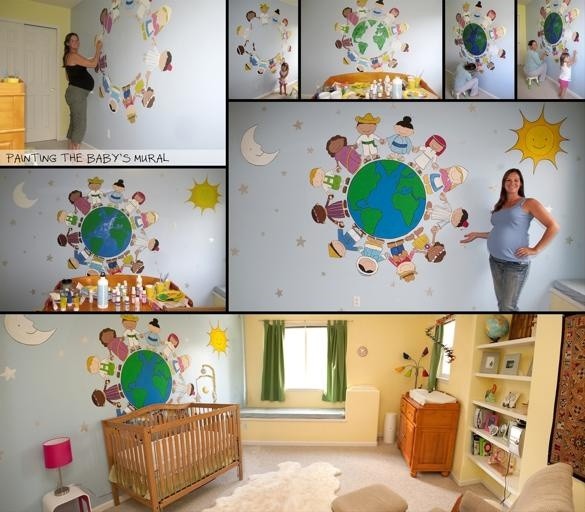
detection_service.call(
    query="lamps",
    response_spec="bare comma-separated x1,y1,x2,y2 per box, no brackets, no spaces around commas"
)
41,436,73,497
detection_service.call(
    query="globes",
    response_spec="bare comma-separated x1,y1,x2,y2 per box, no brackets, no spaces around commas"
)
485,316,509,343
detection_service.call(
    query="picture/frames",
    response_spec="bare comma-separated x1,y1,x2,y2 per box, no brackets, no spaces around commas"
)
501,352,521,375
479,350,501,375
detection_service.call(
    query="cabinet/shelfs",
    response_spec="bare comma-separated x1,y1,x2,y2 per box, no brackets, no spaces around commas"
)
467,314,562,505
211,284,226,308
396,390,461,478
0,78,27,164
547,279,584,312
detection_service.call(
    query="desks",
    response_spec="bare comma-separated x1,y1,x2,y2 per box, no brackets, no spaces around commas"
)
42,275,194,311
163,305,226,311
41,483,93,512
310,71,441,99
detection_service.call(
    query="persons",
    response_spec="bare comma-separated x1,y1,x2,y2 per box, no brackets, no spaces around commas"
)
557,50,578,98
486,384,497,403
452,62,480,98
459,168,560,312
62,33,102,150
278,64,288,96
524,40,547,87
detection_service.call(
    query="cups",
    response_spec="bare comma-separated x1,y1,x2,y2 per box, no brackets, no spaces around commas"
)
58,292,80,312
407,75,421,90
318,91,342,99
62,278,72,290
145,284,157,300
155,279,171,293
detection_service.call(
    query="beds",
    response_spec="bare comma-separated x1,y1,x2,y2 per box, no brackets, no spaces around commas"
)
100,401,244,511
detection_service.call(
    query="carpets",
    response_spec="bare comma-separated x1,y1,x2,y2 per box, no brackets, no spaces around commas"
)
201,460,343,512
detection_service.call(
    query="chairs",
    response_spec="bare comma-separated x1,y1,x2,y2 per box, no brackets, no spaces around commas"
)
329,482,409,512
427,462,575,512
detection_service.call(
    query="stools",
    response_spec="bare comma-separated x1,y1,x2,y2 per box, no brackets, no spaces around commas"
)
451,89,466,99
289,83,298,96
527,76,539,89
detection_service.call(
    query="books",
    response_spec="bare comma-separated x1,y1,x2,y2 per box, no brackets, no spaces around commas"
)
472,434,493,457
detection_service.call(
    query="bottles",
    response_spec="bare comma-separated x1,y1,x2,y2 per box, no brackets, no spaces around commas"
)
112,273,147,310
366,73,391,99
391,74,403,100
88,288,93,303
97,271,109,309
